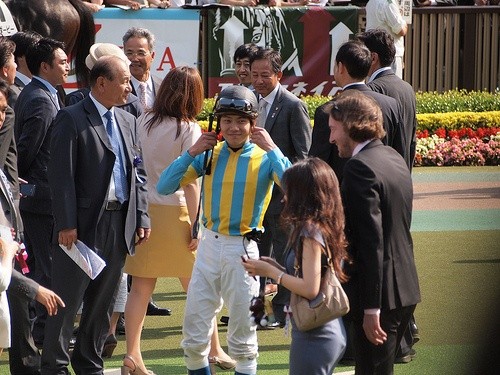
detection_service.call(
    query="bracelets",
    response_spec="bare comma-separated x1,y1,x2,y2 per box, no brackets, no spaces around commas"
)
276,272,285,284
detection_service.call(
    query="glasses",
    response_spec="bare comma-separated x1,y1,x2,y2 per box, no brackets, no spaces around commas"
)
125,50,153,60
331,99,343,121
215,97,252,113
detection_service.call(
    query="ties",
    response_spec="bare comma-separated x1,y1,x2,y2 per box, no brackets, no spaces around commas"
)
255,98,269,129
56,91,65,109
139,82,149,114
105,111,131,204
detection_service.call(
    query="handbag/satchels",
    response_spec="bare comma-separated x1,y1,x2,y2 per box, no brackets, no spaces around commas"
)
290,220,350,332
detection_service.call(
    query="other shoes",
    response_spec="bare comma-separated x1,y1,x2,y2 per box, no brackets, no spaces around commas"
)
410,322,420,344
395,349,412,363
145,300,172,315
115,313,145,334
219,299,288,331
68,337,78,348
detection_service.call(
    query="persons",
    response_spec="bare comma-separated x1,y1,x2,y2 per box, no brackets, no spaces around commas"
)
413,0,500,92
220,43,311,329
81,0,328,13
366,0,409,80
310,28,419,363
242,158,347,375
324,94,422,375
157,85,295,375
121,67,236,375
0,27,164,375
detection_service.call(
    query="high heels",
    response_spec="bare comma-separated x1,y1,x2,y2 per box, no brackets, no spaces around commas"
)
208,355,236,375
102,334,118,359
69,322,80,338
121,354,153,375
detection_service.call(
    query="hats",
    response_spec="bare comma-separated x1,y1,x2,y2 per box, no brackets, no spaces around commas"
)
84,42,132,71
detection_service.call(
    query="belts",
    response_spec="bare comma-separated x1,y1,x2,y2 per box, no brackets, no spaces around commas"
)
105,201,127,211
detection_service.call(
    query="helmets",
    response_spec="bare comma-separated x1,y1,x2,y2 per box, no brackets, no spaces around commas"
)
214,84,259,119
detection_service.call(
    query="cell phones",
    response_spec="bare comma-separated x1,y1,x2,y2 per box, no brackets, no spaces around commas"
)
241,254,259,281
